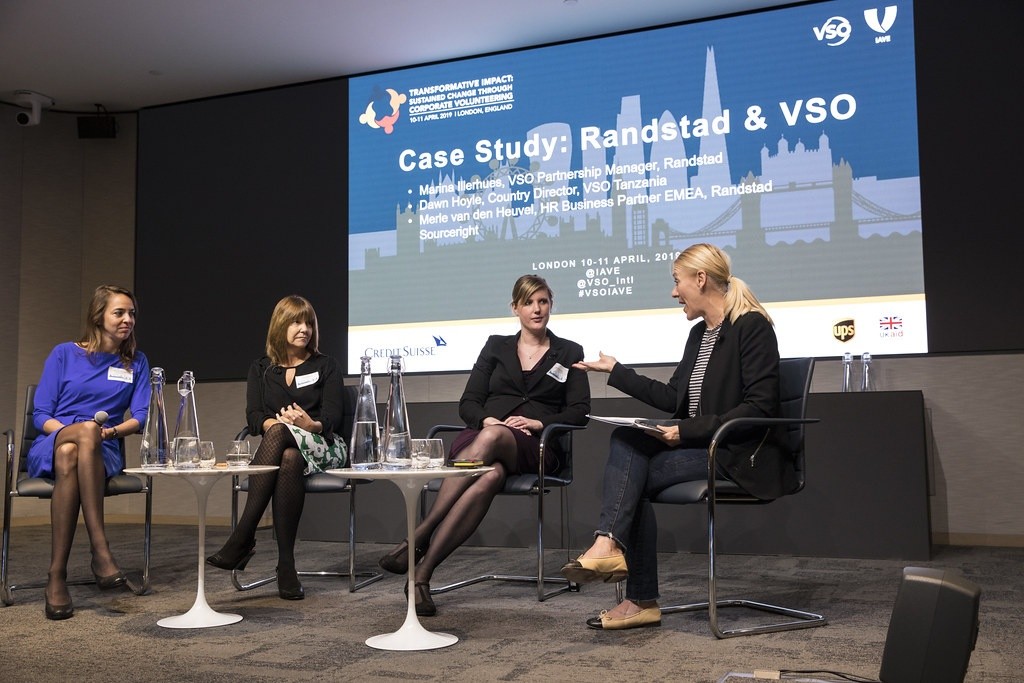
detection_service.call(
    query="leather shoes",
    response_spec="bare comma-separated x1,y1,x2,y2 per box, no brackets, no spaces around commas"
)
561,555,629,583
585,602,661,630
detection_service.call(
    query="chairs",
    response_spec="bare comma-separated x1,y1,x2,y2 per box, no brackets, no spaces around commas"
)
420,423,587,602
232,384,383,593
616,357,827,639
0,385,153,606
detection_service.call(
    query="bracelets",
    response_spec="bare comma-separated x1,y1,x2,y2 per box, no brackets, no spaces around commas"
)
317,421,323,434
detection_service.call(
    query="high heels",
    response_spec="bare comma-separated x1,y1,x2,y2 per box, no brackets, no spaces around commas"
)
404,578,436,617
44,586,74,621
275,566,305,601
91,562,127,592
205,539,256,570
377,539,430,575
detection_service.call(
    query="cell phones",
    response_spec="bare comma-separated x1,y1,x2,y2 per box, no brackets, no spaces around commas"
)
450,458,483,466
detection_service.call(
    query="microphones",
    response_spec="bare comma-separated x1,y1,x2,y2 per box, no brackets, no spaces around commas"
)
91,411,109,428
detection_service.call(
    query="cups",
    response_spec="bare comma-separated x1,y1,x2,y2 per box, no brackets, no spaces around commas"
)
225,440,252,467
168,437,216,470
385,435,444,468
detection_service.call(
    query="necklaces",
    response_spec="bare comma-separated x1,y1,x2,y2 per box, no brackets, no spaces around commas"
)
706,321,718,343
517,337,548,360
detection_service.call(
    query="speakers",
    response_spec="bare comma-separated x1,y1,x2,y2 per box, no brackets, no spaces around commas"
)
76,116,117,140
880,565,981,683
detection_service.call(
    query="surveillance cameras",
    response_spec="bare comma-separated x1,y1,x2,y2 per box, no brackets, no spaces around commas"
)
16,112,32,126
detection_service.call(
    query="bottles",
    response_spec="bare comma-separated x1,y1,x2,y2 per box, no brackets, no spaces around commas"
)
349,356,382,471
860,352,876,392
140,367,170,470
842,353,858,392
381,355,411,445
173,371,199,438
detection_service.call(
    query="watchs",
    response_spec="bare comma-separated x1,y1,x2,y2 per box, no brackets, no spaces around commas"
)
112,426,119,439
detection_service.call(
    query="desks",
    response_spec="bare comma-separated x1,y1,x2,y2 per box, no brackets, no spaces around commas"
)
273,390,932,563
326,464,496,651
123,464,280,629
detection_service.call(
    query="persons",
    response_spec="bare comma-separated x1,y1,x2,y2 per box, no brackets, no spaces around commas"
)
206,295,348,601
27,283,160,621
378,274,592,617
561,243,801,630
